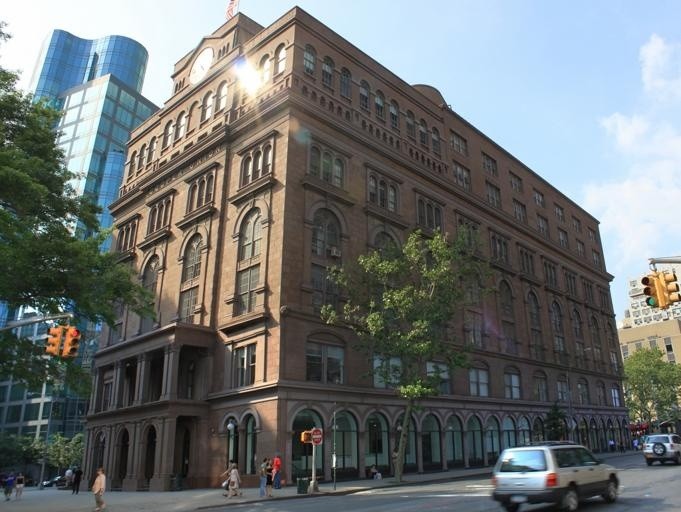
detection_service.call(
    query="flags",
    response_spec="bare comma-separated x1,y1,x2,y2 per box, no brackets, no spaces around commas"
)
225,0,238,20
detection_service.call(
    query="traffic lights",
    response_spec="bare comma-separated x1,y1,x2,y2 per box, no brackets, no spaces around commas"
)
60,326,82,359
299,431,311,444
638,273,665,311
42,325,63,358
657,269,680,307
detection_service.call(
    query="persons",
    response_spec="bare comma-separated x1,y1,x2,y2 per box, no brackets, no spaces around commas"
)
368,463,378,479
91,467,107,511
2,471,16,501
620,442,626,453
272,456,283,489
64,464,84,495
225,462,242,499
220,459,239,496
609,438,616,452
265,458,274,497
633,437,639,450
259,457,267,497
15,471,26,500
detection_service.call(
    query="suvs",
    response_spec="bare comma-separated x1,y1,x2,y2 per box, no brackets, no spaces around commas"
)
488,440,622,512
640,433,681,467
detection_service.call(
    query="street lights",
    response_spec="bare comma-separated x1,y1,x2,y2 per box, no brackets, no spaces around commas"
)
23,390,54,492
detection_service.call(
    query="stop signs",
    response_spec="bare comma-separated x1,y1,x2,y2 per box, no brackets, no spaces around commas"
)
310,428,323,446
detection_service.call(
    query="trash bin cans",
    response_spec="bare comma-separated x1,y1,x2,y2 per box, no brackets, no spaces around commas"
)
296,475,309,494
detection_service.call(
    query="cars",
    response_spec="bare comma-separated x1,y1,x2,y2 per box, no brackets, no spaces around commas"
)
41,475,66,488
640,434,654,441
